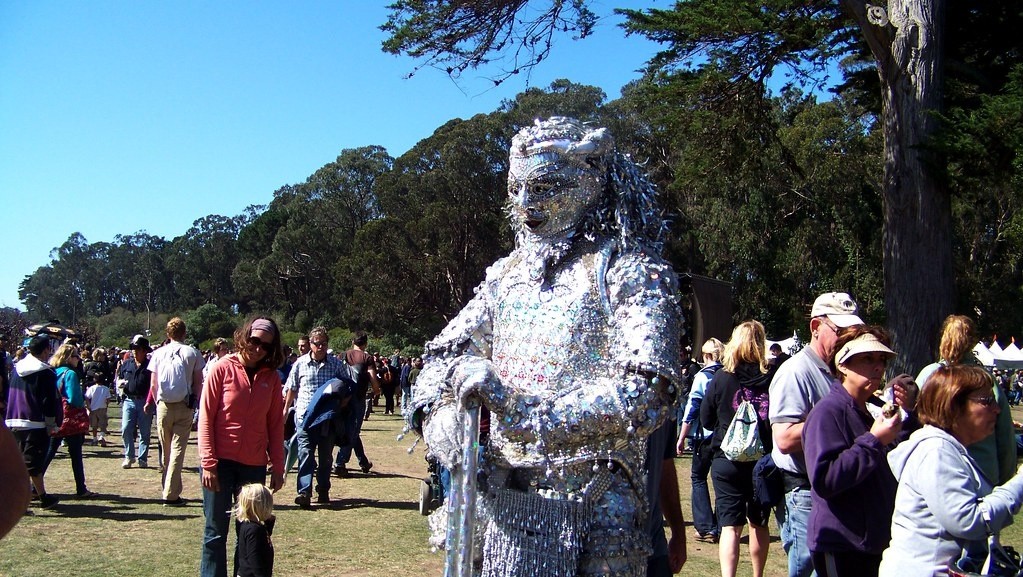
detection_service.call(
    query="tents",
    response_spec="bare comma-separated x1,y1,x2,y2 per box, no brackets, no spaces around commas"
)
989,341,1002,356
971,341,997,371
994,343,1023,371
764,330,800,365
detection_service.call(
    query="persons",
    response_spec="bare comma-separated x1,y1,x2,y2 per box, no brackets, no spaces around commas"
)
282,326,352,506
198,315,285,577
404,116,685,577
226,483,276,577
0,416,36,540
0,332,424,480
648,292,1023,577
31,343,99,498
5,335,60,510
147,317,205,508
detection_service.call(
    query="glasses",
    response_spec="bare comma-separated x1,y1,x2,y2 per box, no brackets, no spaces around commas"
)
967,394,996,405
72,355,79,359
818,317,845,336
313,340,328,346
249,336,271,352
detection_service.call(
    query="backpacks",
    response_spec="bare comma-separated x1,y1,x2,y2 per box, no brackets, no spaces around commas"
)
720,368,765,462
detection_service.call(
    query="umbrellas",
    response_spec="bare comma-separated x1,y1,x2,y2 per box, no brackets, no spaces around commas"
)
23,322,76,352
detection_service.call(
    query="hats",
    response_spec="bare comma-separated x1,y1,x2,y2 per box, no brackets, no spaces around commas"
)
810,292,866,328
129,334,153,353
835,332,898,369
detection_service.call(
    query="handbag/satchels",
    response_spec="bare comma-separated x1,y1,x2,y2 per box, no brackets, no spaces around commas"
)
343,352,372,392
753,453,776,506
49,369,90,437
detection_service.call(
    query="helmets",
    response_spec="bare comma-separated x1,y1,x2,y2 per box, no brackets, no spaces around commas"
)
946,530,1022,577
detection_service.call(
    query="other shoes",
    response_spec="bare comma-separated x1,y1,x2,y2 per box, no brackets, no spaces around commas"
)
40,497,59,509
91,441,98,445
98,437,106,447
362,461,373,472
334,466,348,475
122,457,136,469
318,493,331,503
76,488,98,498
294,492,311,507
163,497,186,506
694,530,715,542
